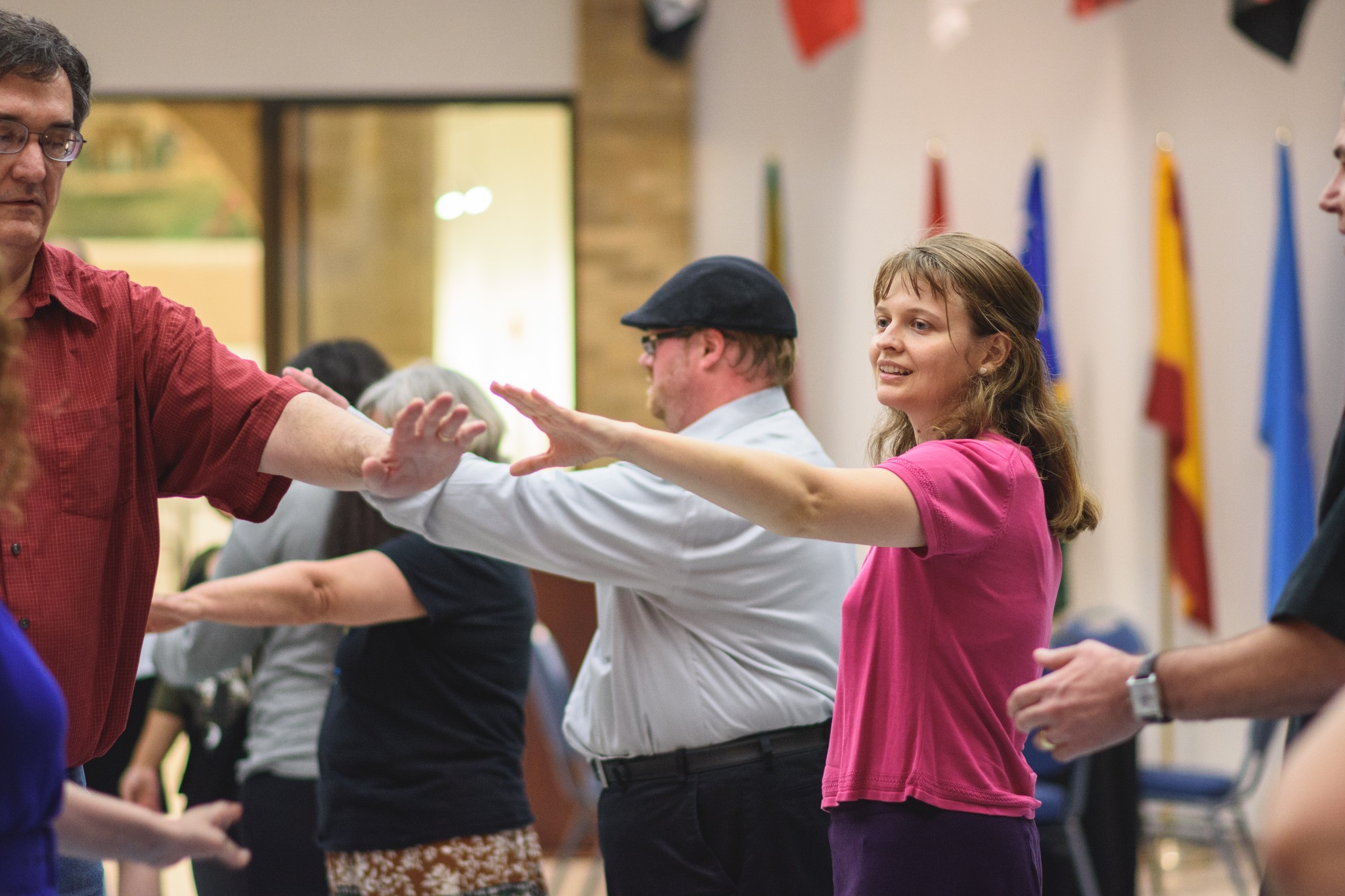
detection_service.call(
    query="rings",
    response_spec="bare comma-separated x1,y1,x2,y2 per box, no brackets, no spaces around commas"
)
435,432,456,442
1036,730,1056,752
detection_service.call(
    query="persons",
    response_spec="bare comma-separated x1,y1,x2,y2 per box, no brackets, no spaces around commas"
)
0,3,552,895
491,233,1108,896
1006,122,1345,896
273,259,863,896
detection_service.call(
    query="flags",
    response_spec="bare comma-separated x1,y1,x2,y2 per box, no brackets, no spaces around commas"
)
1005,158,1069,617
1247,137,1314,621
918,156,952,236
1134,127,1219,628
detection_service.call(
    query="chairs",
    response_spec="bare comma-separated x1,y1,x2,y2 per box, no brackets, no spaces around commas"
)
1015,614,1278,896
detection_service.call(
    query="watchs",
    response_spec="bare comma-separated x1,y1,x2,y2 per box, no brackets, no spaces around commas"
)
1129,645,1174,720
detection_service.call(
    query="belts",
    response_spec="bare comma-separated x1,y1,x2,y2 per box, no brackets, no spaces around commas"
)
592,722,824,789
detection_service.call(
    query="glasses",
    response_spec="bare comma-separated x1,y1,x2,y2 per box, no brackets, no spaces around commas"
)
0,119,87,162
640,328,738,357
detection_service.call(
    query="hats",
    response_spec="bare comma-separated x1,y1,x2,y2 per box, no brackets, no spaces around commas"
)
621,256,798,338
